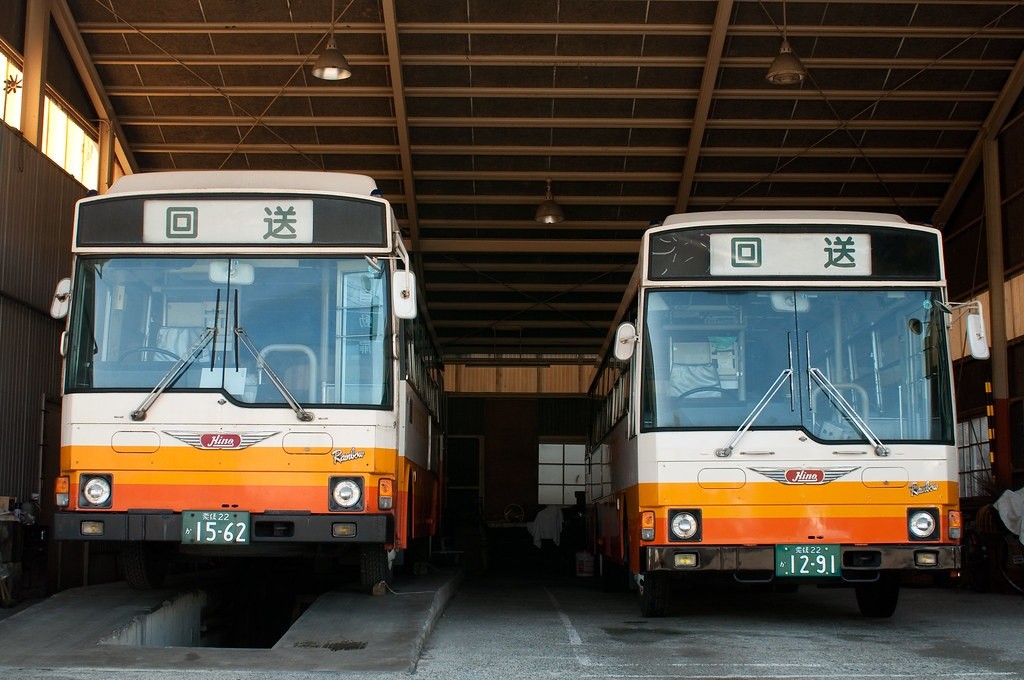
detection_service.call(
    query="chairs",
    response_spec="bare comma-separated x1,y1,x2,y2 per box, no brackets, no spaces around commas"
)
151,325,211,363
664,362,723,402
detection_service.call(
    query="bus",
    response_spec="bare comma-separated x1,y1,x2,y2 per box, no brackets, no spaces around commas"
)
44,166,446,595
581,208,991,622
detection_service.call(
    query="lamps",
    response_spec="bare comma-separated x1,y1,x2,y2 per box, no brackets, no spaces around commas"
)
311,0,352,81
765,1,809,85
534,177,566,224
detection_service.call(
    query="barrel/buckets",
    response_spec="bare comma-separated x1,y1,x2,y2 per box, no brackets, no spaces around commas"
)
575,552,593,578
